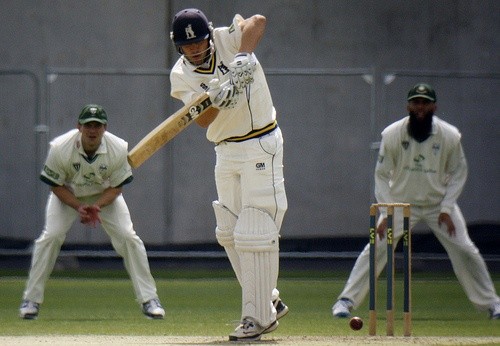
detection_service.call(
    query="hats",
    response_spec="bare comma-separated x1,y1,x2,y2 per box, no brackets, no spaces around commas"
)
407,83,436,102
78,105,108,125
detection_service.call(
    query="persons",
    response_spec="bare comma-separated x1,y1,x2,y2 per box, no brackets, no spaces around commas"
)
332,83,500,319
20,105,165,319
170,8,289,342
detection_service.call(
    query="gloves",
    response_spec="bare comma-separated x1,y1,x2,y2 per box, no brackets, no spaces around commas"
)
228,52,255,94
207,78,238,110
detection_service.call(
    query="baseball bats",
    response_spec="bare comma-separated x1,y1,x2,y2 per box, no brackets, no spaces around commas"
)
126,78,235,168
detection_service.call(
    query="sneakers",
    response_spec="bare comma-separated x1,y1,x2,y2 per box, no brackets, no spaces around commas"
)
488,304,500,319
19,301,39,319
141,299,165,318
272,296,289,320
331,299,353,317
228,318,279,341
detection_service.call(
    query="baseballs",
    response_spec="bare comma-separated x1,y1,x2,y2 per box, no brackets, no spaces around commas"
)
350,317,362,330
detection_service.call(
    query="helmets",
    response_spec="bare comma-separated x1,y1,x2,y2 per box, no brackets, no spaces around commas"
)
170,7,213,54
